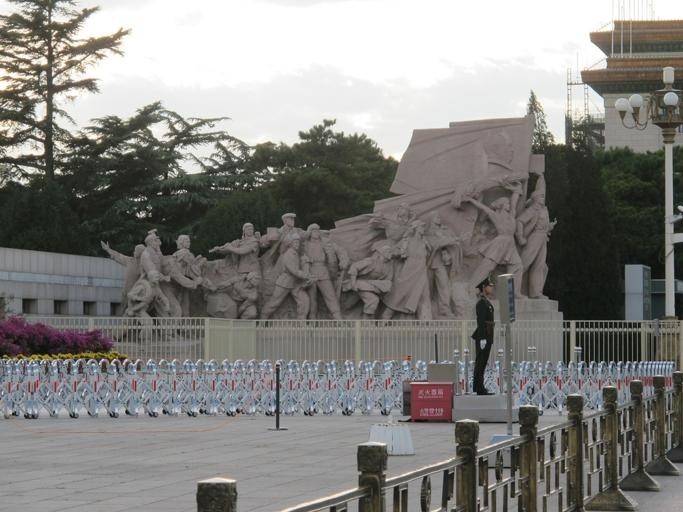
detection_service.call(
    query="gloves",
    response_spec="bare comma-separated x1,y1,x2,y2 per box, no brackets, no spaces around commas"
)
480,339,487,350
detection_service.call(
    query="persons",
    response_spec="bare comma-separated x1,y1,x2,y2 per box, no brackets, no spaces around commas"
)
461,180,528,300
515,190,554,299
100,230,206,344
201,202,463,328
469,277,496,396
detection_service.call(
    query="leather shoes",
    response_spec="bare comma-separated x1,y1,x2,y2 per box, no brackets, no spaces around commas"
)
472,391,496,396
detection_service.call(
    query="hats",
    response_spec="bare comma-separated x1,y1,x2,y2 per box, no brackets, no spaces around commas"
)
474,276,495,291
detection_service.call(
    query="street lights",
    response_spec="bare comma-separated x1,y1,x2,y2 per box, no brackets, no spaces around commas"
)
615,66,682,370
